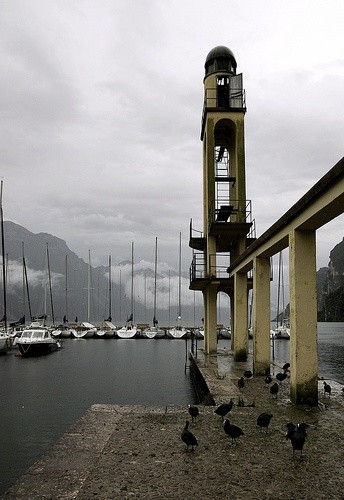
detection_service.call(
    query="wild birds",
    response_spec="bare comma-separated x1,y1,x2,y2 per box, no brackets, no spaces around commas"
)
214,400,234,420
269,383,278,398
188,404,199,423
264,377,273,384
182,421,198,451
276,371,288,381
285,422,308,457
282,362,291,370
322,381,331,394
256,413,273,432
238,377,245,389
244,371,253,378
222,419,244,445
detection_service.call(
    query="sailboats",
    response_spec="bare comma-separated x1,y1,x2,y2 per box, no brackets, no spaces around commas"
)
166,232,231,339
249,251,290,339
45,242,73,337
0,180,63,357
93,254,117,338
139,237,166,339
112,241,141,339
71,248,97,338
